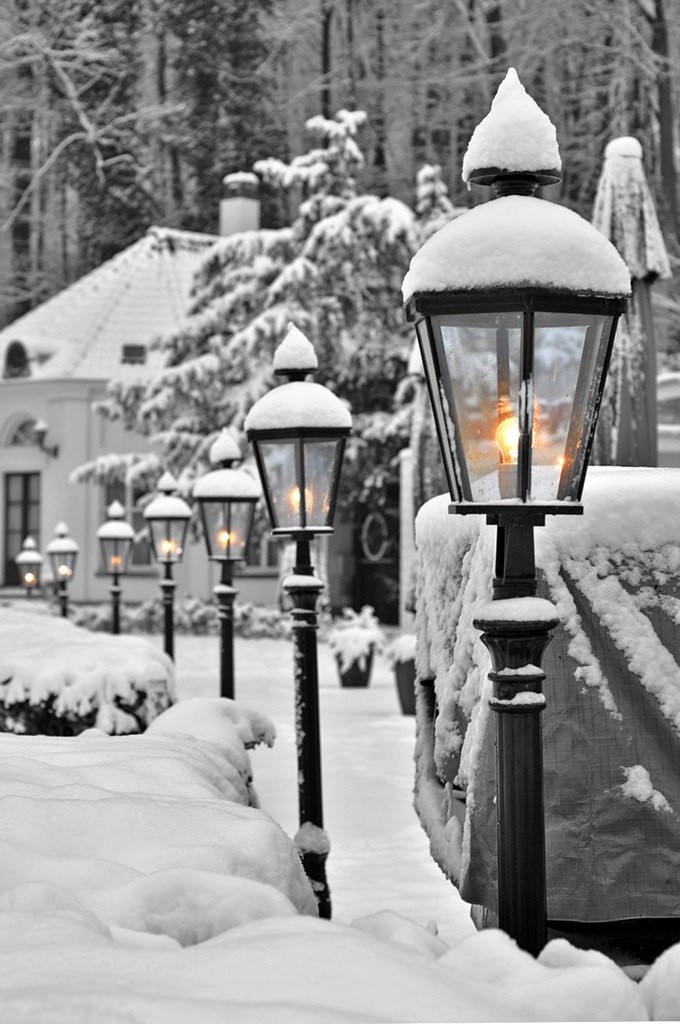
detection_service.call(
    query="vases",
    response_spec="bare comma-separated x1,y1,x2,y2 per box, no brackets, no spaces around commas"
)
330,642,374,688
391,657,418,716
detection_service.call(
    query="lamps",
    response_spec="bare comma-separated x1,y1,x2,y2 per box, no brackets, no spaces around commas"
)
33,418,58,459
